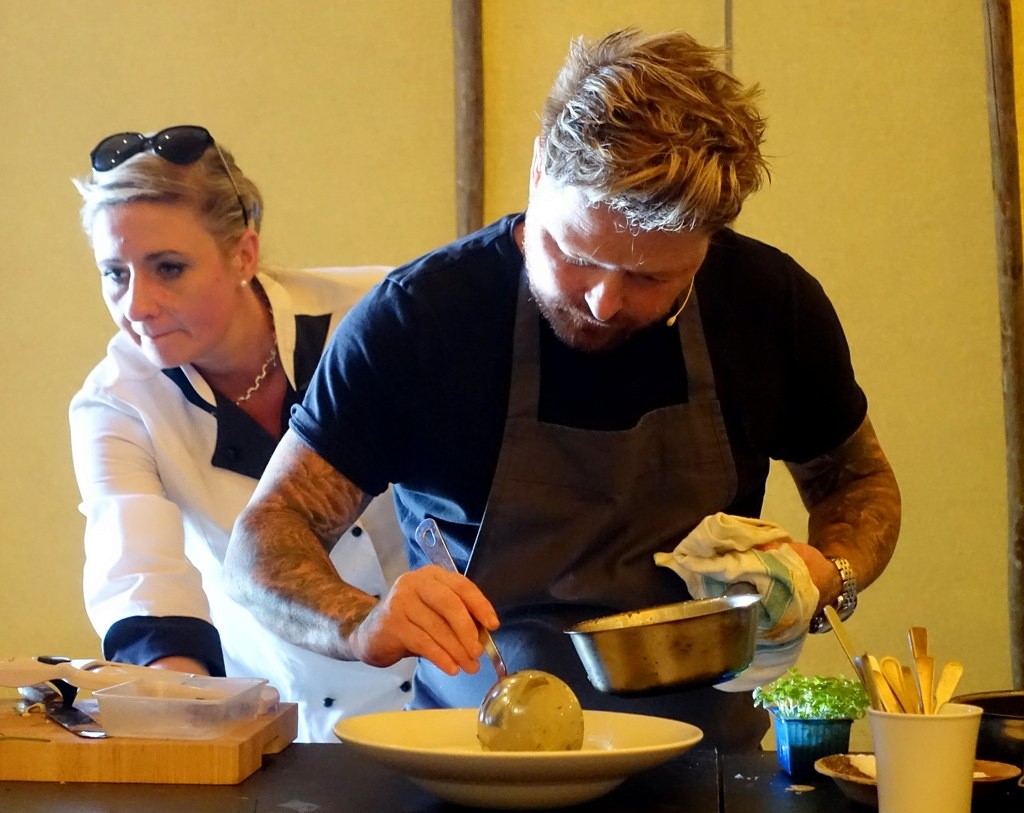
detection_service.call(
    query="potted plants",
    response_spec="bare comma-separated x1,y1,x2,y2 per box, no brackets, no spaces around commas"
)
753,667,873,777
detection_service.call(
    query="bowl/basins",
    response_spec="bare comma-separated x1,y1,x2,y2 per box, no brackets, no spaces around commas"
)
334,707,705,809
949,688,1023,772
813,752,1021,803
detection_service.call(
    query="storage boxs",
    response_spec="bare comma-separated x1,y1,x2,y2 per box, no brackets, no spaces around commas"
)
92,673,268,741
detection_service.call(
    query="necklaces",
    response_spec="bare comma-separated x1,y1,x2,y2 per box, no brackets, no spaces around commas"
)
234,298,277,404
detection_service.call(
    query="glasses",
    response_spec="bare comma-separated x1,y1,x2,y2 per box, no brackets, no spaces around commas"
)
89,126,250,230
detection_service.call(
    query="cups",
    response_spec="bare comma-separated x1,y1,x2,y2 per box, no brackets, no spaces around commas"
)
867,702,983,811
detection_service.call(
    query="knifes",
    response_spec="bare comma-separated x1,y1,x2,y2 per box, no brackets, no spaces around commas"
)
42,703,109,740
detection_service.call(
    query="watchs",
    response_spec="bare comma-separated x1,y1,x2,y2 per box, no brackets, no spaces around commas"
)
810,555,859,635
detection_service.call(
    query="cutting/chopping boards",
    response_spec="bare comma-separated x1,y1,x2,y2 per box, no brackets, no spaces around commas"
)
0,663,300,787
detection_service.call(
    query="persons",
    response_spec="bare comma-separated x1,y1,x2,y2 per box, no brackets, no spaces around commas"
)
220,24,902,755
66,124,419,745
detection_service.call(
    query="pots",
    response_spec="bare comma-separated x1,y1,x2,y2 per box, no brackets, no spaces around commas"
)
560,582,765,692
